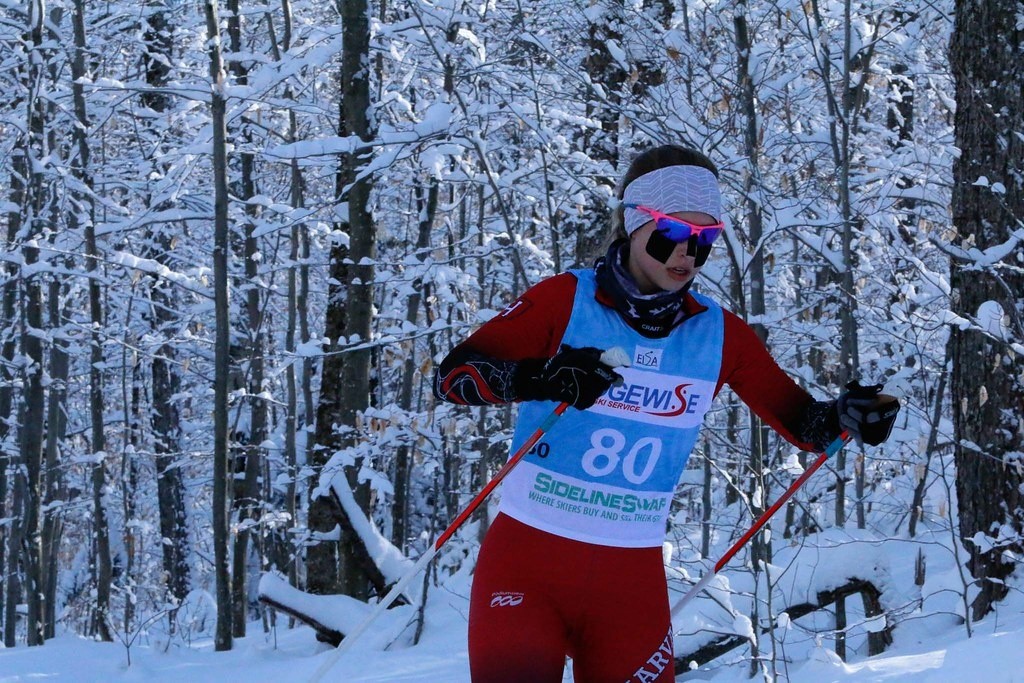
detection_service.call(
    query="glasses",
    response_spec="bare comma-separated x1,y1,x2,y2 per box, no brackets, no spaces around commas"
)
622,203,726,245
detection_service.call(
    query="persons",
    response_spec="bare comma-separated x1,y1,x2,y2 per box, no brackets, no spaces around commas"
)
432,144,901,683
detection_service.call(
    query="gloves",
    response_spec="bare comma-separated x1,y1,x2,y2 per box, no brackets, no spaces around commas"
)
839,394,899,446
515,344,619,411
